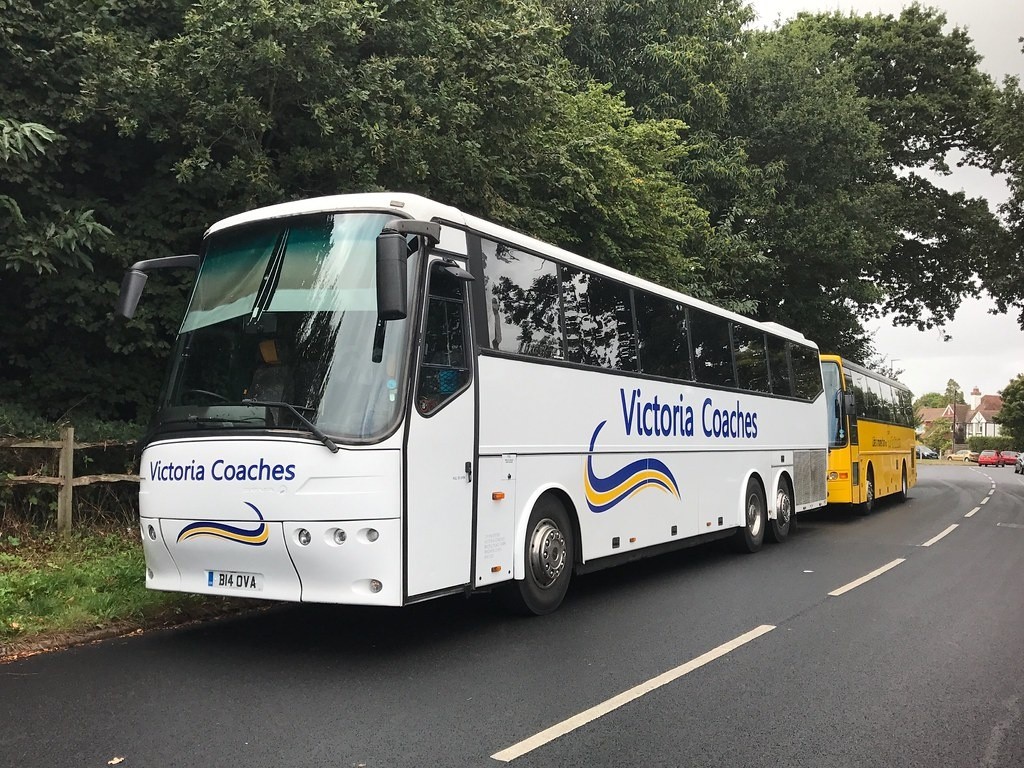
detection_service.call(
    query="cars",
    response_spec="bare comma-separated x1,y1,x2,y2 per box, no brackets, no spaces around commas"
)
1000,451,1020,465
1014,453,1024,474
978,450,1006,467
916,445,938,459
947,449,979,462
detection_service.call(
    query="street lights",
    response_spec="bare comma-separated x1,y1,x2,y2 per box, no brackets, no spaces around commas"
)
891,359,901,376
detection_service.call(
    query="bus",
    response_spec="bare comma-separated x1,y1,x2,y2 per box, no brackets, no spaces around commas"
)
735,355,917,515
111,190,830,616
